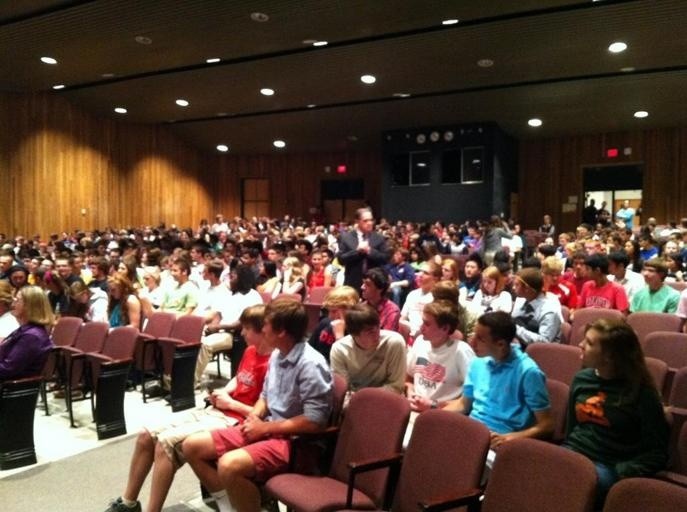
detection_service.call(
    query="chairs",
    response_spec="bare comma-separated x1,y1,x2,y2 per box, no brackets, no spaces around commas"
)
180,308,687,511
1,284,331,469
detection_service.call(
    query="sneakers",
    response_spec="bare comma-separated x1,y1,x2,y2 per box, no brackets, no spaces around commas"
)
103,498,142,512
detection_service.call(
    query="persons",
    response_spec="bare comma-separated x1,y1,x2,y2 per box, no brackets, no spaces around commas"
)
0,197,109,382
103,198,686,511
